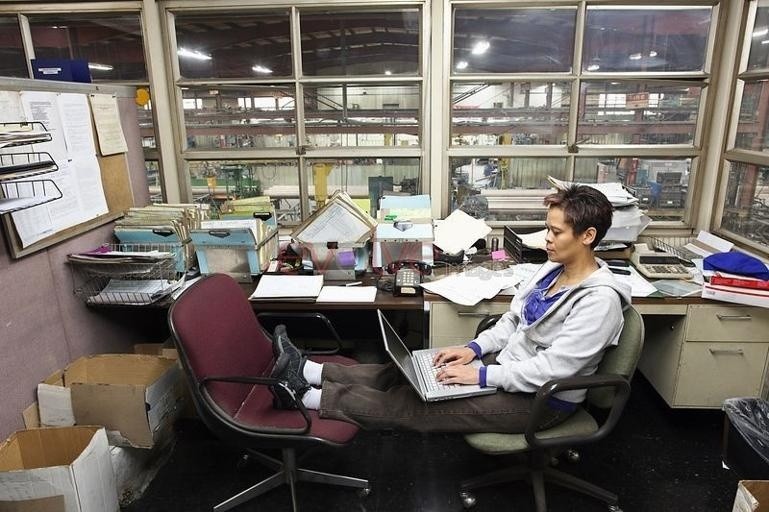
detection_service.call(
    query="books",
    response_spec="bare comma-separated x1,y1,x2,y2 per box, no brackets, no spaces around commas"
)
67,173,659,309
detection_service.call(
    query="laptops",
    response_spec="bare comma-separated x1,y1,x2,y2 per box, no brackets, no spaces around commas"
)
376,306,498,405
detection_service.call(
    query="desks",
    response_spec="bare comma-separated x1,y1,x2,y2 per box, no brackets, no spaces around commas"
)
88,256,425,316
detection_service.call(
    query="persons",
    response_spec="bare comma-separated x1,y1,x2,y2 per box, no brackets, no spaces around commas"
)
265,183,623,436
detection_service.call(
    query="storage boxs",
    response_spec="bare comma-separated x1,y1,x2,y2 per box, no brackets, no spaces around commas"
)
1,343,187,512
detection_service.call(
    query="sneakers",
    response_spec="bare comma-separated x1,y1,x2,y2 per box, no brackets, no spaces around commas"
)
272,325,308,385
268,352,311,409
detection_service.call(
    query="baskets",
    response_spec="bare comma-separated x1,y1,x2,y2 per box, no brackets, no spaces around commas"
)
68,243,184,303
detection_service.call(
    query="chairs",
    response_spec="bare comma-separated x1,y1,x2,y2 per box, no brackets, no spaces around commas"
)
462,303,646,512
167,275,373,511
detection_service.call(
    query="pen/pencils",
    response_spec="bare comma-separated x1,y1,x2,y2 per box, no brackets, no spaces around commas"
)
339,281,362,286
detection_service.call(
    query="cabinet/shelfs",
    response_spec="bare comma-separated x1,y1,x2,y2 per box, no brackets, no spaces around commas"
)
639,304,769,446
428,303,512,349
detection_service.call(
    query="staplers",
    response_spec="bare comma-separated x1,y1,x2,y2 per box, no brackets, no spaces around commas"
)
606,262,630,275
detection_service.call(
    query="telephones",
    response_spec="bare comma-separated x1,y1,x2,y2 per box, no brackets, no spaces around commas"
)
388,258,432,297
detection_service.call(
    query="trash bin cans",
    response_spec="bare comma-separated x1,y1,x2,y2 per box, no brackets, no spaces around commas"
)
722,397,769,481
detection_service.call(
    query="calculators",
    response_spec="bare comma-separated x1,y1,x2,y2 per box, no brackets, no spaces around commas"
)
637,251,694,279
